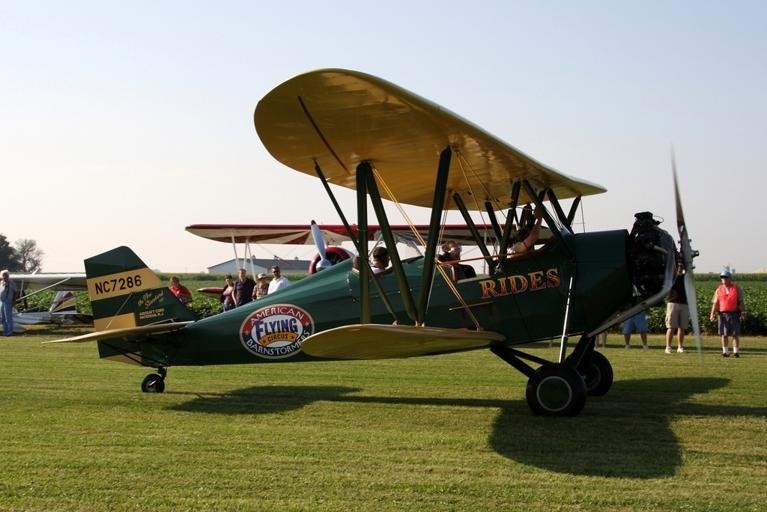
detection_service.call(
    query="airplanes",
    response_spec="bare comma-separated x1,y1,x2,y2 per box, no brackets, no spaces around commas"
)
40,65,708,419
0,271,94,328
178,218,557,300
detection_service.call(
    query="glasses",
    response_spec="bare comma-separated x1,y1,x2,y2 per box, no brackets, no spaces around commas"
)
226,278,234,283
272,270,280,274
677,264,684,268
448,246,463,254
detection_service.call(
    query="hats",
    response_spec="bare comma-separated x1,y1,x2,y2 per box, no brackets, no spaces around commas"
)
257,273,269,281
720,271,731,278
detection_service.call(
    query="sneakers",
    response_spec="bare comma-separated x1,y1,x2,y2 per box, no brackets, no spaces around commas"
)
594,343,608,350
624,344,632,350
677,345,684,353
2,331,15,337
733,353,740,358
721,352,730,357
665,346,673,353
643,345,648,351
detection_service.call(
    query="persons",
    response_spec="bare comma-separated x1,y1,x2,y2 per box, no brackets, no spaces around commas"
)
664,260,690,354
709,271,749,358
352,240,476,289
252,273,270,301
0,269,18,336
622,310,648,350
167,276,192,305
223,274,239,312
232,270,257,307
267,266,289,295
